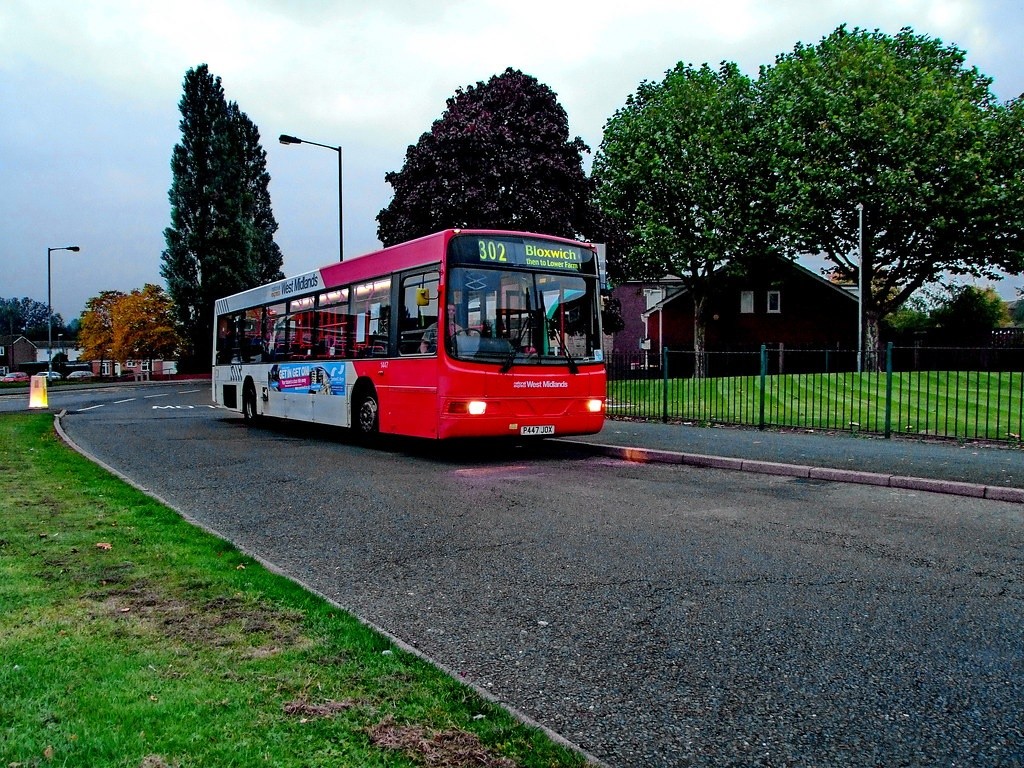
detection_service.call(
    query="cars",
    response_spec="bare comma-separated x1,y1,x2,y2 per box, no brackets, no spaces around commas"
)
1,372,30,381
36,371,63,380
67,371,94,379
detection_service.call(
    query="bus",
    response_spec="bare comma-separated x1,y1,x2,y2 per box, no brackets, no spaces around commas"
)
212,229,608,449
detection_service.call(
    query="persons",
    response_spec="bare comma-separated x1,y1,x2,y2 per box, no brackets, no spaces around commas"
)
421,302,465,353
250,329,335,356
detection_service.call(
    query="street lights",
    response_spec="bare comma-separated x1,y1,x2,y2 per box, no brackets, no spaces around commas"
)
278,134,344,262
47,246,81,385
